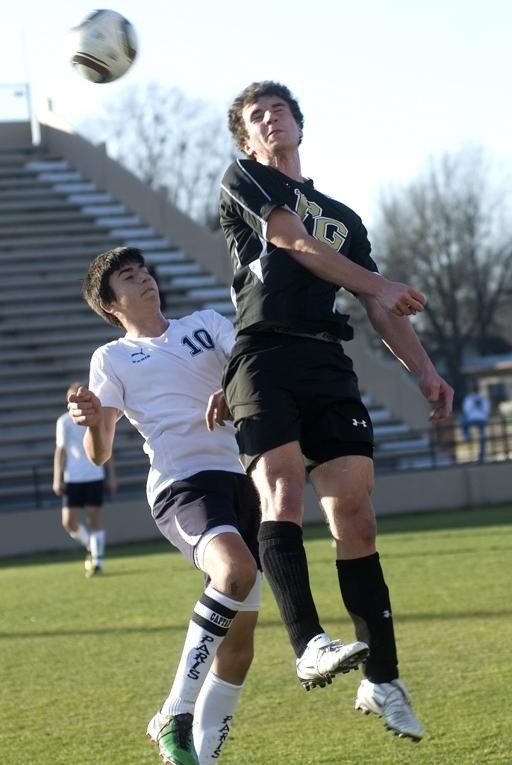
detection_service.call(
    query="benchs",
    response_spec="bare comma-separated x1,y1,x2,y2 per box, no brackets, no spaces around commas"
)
0,145,433,512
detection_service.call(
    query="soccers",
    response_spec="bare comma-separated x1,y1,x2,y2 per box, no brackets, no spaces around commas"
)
67,8,136,83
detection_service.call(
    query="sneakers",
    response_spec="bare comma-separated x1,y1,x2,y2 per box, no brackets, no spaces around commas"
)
145,708,200,765
85,553,101,578
356,678,423,742
295,632,371,691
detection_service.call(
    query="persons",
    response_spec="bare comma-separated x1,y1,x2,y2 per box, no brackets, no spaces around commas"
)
52,382,116,578
220,79,458,747
67,248,257,764
460,383,492,464
